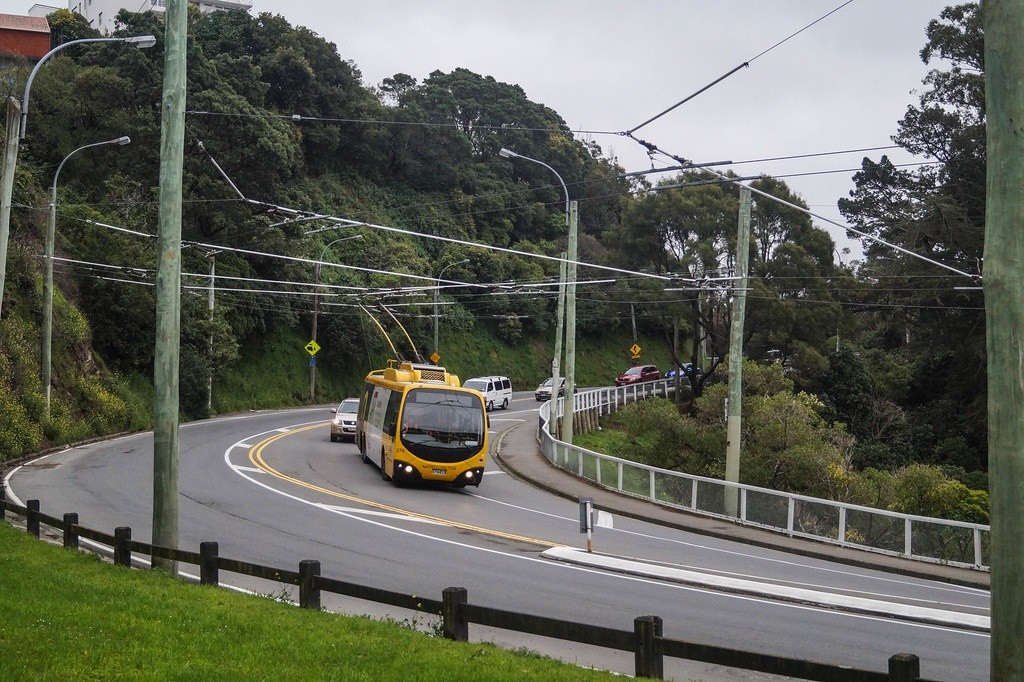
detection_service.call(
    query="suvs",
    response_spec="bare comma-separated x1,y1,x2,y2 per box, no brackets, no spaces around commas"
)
615,365,660,390
331,398,360,442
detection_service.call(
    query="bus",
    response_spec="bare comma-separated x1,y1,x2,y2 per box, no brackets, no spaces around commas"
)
355,359,490,489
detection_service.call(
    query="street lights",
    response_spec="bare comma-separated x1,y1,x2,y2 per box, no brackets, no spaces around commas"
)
498,147,577,446
429,259,469,366
0,35,155,318
40,136,131,418
304,235,362,401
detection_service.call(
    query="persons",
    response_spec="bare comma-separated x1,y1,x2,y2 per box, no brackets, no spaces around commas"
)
404,415,422,432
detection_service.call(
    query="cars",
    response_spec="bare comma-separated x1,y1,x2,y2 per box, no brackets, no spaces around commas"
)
535,377,577,401
665,363,701,384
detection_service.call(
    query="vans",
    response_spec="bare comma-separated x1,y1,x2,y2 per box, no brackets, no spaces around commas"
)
462,376,512,411
765,350,784,363
